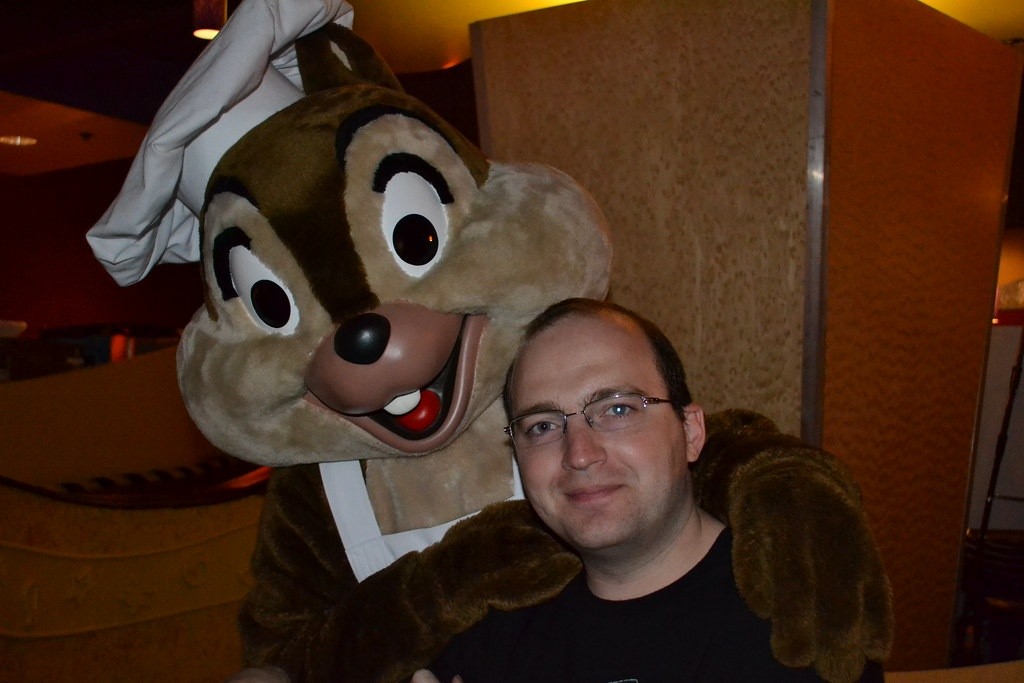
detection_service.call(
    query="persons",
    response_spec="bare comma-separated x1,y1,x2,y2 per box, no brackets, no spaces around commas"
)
411,297,885,682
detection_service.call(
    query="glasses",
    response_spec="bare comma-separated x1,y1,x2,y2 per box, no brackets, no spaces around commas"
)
503,393,680,449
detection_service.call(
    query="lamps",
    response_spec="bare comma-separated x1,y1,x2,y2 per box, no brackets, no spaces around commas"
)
192,0,228,39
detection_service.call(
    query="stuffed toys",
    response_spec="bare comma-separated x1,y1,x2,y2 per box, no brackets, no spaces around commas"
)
86,0,895,682
